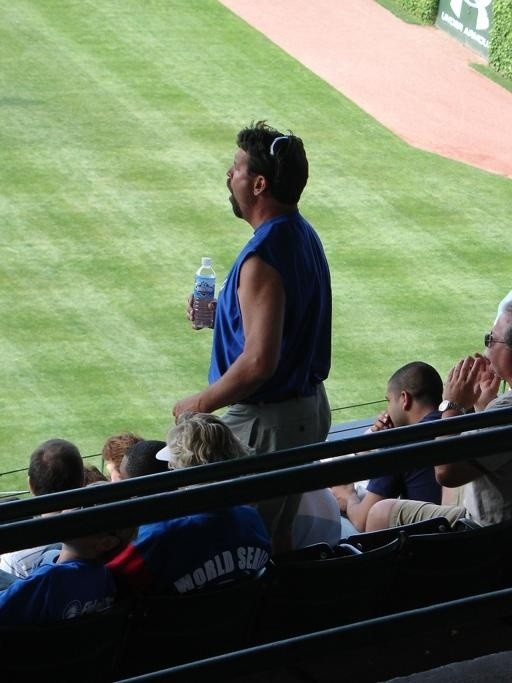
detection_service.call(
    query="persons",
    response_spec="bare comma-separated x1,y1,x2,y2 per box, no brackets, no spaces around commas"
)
0,413,272,623
345,361,443,535
290,488,341,550
172,119,336,563
366,292,512,530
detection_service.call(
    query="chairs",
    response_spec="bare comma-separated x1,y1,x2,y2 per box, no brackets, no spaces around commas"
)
275,542,338,564
345,515,455,553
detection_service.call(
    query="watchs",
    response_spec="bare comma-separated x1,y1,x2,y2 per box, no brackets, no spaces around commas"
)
439,400,465,413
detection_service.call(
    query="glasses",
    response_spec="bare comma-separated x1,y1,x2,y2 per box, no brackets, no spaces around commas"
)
484,333,509,347
270,135,290,189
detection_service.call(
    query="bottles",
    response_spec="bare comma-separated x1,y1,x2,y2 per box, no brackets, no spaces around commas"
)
192,256,216,328
